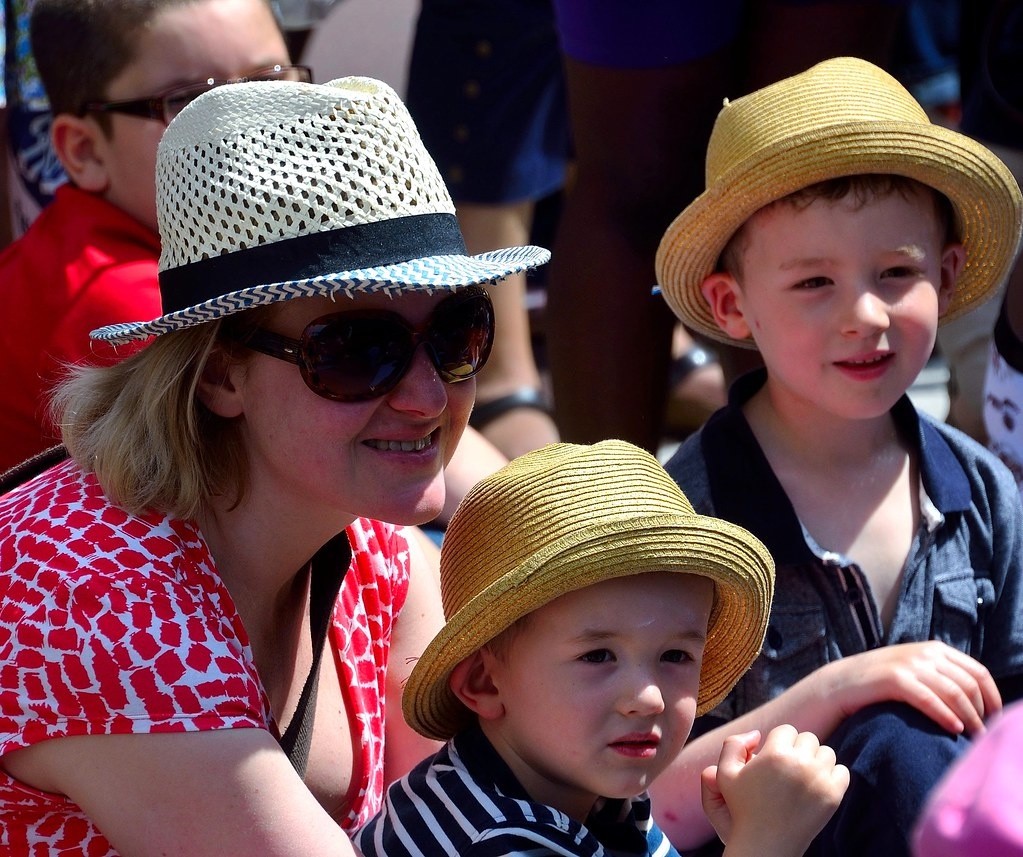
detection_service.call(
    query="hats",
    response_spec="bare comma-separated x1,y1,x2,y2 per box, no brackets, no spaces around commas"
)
89,78,552,348
402,439,777,743
652,56,1023,350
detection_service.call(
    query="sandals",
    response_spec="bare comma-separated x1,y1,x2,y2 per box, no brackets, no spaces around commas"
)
470,387,558,459
620,338,728,446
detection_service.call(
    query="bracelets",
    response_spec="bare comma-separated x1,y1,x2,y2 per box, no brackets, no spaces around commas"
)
668,345,716,393
469,390,552,428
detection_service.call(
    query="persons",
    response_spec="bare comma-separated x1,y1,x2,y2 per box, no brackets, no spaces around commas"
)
0,78,551,856
351,440,850,856
666,56,1023,856
2,0,1022,549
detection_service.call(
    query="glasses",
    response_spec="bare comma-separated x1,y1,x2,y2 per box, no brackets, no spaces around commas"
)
75,64,314,130
219,285,495,403
